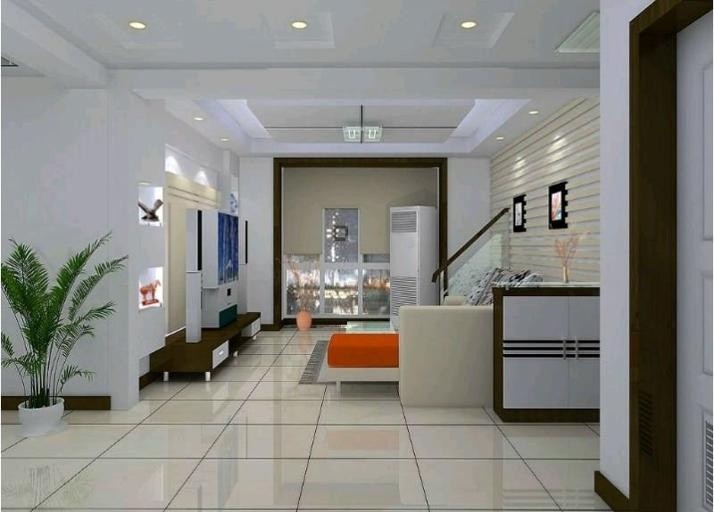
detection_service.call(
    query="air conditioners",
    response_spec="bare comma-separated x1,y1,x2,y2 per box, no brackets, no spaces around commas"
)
386,206,441,334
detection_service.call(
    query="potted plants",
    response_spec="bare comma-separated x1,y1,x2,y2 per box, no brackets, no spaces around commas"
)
0,230,131,438
556,230,591,283
289,265,317,330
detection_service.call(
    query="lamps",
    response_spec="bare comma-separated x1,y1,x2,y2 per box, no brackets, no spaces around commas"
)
343,126,383,143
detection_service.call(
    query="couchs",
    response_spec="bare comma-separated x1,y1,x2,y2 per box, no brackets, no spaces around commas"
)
394,267,567,407
328,332,402,391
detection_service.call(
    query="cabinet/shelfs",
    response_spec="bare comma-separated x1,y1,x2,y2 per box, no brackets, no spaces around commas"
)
150,311,261,383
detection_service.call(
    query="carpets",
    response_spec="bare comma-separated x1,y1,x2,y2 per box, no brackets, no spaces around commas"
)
298,339,338,384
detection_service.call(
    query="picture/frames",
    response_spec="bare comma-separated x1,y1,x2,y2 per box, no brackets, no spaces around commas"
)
547,181,568,228
512,195,527,232
